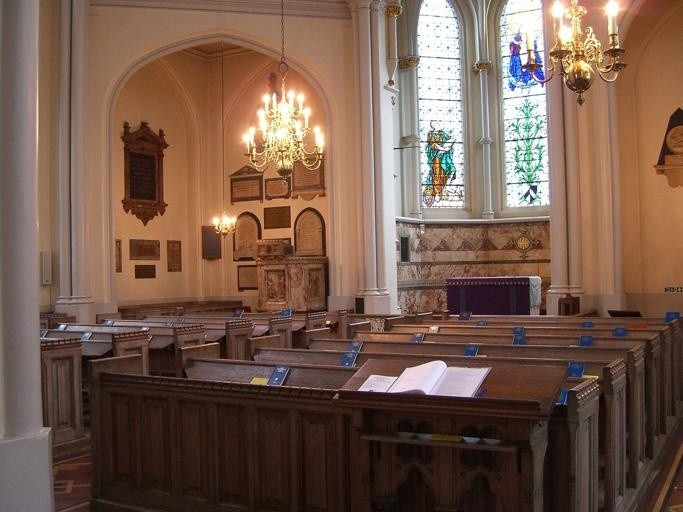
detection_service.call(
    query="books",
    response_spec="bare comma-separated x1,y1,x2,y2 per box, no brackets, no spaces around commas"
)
664,311,680,323
40,330,47,338
164,321,173,326
346,341,363,352
577,335,593,347
279,306,291,317
511,326,524,336
56,324,66,330
408,332,425,342
80,332,92,340
106,320,114,324
473,319,486,326
140,327,148,330
232,309,244,318
385,359,493,397
174,316,184,323
266,365,290,385
566,361,584,377
613,327,626,337
341,352,357,367
428,325,439,333
457,310,472,320
582,321,592,328
511,335,527,345
462,344,479,357
554,387,569,405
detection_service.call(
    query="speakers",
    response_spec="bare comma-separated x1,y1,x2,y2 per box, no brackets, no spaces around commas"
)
42,251,52,285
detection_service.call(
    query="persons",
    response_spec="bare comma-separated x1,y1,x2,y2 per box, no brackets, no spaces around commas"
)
423,117,458,203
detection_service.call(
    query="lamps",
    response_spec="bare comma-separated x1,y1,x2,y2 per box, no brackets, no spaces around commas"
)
240,1,327,183
508,1,629,106
